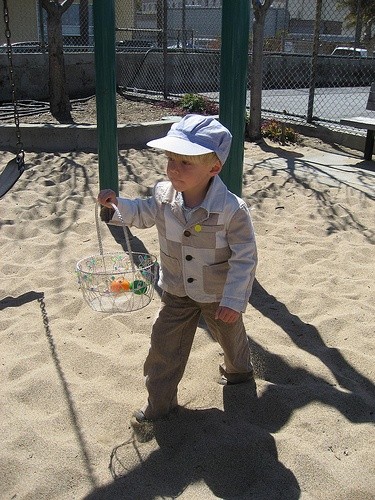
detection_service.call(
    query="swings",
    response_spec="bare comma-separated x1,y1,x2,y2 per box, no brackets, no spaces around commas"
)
0,0,25,197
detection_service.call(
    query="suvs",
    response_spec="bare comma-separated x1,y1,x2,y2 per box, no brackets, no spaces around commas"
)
114,40,160,51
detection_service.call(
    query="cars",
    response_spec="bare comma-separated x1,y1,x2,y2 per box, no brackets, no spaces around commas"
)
2,40,46,47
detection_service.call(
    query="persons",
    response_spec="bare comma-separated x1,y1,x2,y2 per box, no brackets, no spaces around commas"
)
97,113,259,424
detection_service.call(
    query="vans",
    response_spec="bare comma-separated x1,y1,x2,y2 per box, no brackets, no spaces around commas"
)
167,36,219,51
330,47,368,58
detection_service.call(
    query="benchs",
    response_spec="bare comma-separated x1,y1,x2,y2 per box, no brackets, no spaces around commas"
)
339,116,374,161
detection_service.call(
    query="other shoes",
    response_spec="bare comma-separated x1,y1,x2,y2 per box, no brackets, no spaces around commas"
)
217,376,252,385
133,410,147,423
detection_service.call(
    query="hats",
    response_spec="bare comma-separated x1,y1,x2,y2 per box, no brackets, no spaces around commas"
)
146,114,233,167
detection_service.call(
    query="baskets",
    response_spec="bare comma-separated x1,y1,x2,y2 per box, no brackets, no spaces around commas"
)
76,201,157,313
